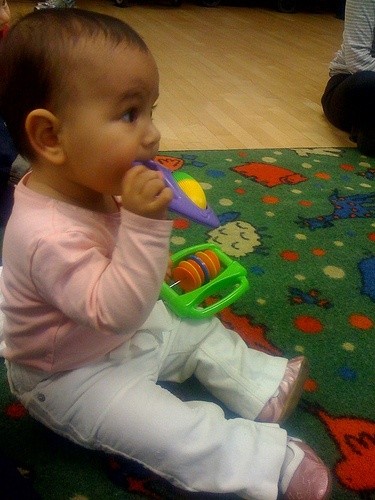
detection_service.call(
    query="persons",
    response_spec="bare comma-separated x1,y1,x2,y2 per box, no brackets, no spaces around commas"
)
321,1,375,154
0,0,333,500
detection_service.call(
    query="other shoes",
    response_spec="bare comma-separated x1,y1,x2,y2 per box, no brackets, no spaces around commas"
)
257,356,308,429
279,434,333,500
